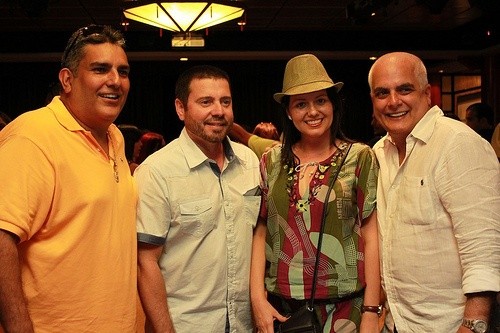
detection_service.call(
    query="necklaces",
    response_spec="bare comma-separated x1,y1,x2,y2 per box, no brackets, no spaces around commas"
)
89,129,119,184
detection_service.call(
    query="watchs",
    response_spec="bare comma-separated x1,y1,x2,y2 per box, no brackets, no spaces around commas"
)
360,304,383,317
462,317,489,333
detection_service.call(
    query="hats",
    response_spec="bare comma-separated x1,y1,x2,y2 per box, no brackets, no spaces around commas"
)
274,54,344,103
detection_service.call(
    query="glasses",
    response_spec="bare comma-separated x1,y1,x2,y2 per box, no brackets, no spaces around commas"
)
64,26,105,59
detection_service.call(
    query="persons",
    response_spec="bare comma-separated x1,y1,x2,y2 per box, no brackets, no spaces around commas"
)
360,110,388,148
228,123,285,161
465,102,494,143
249,54,383,333
136,132,165,178
130,65,263,333
252,121,280,142
367,52,500,333
117,124,143,176
0,23,147,333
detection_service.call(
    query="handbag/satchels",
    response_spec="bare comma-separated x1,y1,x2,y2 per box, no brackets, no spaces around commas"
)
274,302,321,333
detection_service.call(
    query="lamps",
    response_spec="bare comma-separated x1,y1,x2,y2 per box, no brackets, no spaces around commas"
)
122,0,247,40
